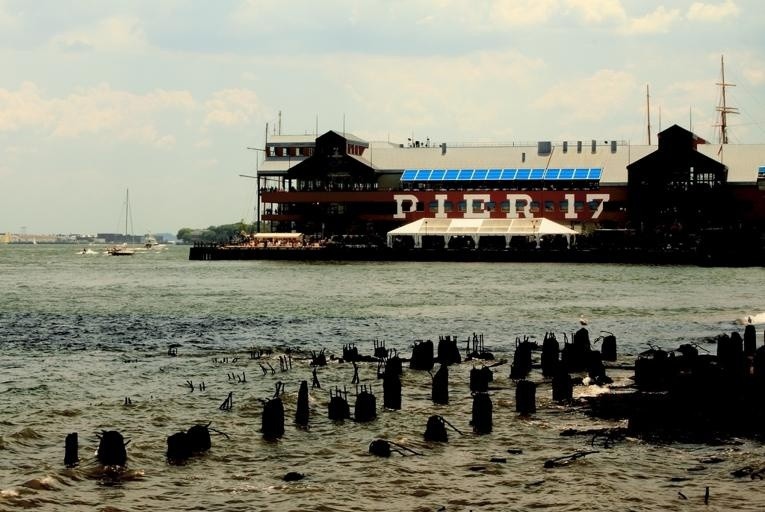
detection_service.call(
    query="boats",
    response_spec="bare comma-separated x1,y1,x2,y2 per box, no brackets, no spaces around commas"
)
143,234,159,249
166,239,176,247
74,247,100,256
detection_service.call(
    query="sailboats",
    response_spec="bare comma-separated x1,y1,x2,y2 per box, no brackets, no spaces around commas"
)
109,184,138,256
